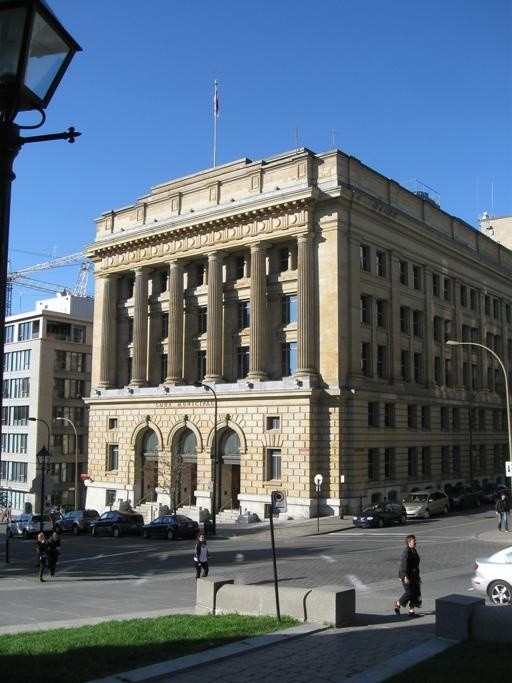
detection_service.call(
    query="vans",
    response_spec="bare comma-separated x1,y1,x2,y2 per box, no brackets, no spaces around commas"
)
401,488,450,519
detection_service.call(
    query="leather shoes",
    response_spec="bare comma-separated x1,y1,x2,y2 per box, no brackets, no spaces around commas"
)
394,600,424,618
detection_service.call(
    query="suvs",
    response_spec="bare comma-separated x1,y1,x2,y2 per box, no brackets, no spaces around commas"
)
5,512,53,538
86,509,144,536
54,509,101,535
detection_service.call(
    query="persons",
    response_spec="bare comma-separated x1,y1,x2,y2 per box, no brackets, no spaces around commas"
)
193,532,211,580
394,534,424,617
45,531,63,577
32,531,49,582
495,493,510,532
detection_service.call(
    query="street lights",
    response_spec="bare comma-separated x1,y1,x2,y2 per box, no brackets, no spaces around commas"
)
192,381,218,534
35,446,51,534
0,2,80,295
55,416,79,511
26,417,50,455
446,341,510,476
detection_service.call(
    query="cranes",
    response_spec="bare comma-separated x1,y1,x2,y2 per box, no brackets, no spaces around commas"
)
3,243,101,324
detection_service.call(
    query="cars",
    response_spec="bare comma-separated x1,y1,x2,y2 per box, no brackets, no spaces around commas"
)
468,545,511,604
353,503,409,529
449,483,484,508
142,514,200,541
482,483,509,504
